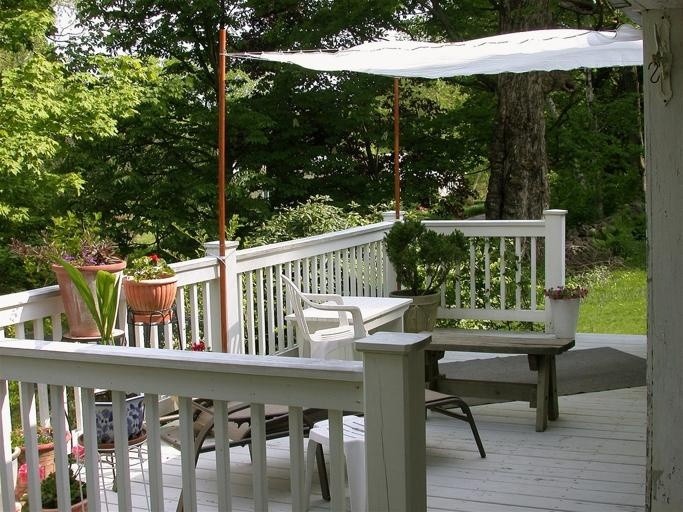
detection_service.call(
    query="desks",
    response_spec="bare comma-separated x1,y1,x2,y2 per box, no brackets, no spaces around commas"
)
287,296,414,333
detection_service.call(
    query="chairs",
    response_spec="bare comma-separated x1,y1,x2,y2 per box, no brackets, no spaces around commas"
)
281,272,371,362
161,385,488,512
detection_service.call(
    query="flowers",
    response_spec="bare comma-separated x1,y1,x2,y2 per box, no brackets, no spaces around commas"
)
6,228,122,273
542,285,588,299
123,254,175,285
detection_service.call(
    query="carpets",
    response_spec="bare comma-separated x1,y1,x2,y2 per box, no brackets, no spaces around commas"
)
434,346,646,410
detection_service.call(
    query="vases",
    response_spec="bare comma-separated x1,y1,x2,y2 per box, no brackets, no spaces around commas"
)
94,388,145,443
550,298,578,339
50,260,127,337
123,275,178,324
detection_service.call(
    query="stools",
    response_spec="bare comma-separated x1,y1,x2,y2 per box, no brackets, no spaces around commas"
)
306,414,368,512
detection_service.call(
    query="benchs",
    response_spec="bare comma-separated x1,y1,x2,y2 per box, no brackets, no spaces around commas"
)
426,328,575,432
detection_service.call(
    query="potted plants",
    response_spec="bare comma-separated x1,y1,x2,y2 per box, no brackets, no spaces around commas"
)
21,468,88,512
382,214,471,332
11,426,71,478
